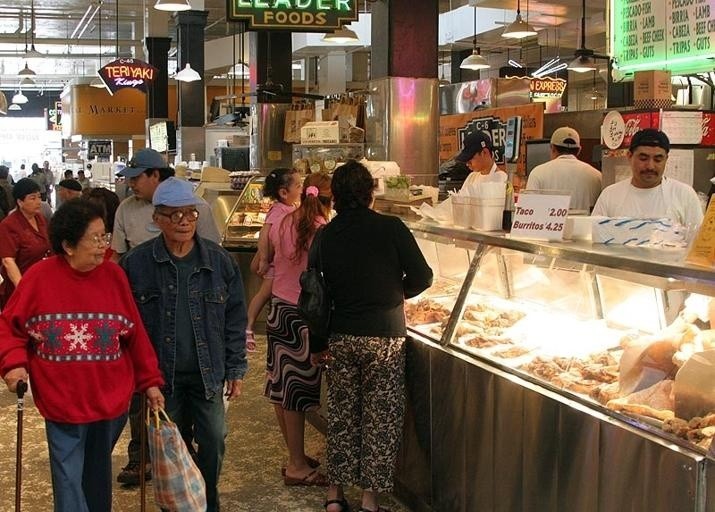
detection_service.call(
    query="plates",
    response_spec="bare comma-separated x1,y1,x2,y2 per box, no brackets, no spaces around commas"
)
401,298,454,330
462,299,528,333
407,275,472,298
602,111,627,152
417,318,486,341
458,333,535,370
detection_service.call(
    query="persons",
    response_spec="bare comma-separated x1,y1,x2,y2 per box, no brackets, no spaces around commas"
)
250,173,335,487
42,162,54,193
58,178,82,201
117,177,249,512
1,178,50,309
454,133,509,190
111,147,224,484
18,165,28,181
27,163,50,200
84,163,93,179
525,128,603,215
0,165,15,193
1,198,165,512
665,292,712,331
590,129,705,229
77,171,90,190
65,170,74,178
303,160,433,511
247,168,302,349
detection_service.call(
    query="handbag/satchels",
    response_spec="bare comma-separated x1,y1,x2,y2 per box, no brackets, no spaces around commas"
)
144,404,208,511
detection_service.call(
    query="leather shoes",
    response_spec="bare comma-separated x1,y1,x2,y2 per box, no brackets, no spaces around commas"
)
118,461,150,482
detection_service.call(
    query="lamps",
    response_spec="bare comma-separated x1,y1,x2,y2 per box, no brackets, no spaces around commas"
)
20,77,36,86
501,0,537,39
18,18,35,74
460,1,491,71
174,10,202,83
153,0,191,12
23,0,44,58
89,0,106,89
12,92,29,104
567,0,597,73
322,26,359,43
8,104,22,110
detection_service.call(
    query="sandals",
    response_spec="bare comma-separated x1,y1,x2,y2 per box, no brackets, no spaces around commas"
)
285,470,328,486
360,505,390,512
281,456,319,475
324,498,348,512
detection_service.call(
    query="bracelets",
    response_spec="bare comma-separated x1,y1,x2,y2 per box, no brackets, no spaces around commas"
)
678,311,690,326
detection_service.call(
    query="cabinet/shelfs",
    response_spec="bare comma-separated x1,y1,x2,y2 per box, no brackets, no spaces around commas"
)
304,207,714,511
188,174,272,335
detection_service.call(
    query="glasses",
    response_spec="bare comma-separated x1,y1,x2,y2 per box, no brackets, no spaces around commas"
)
90,233,112,248
159,210,199,223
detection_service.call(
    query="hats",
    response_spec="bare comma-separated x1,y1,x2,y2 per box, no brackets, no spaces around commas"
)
630,128,670,152
551,126,580,148
60,179,82,191
152,177,207,207
116,148,167,177
457,133,490,161
